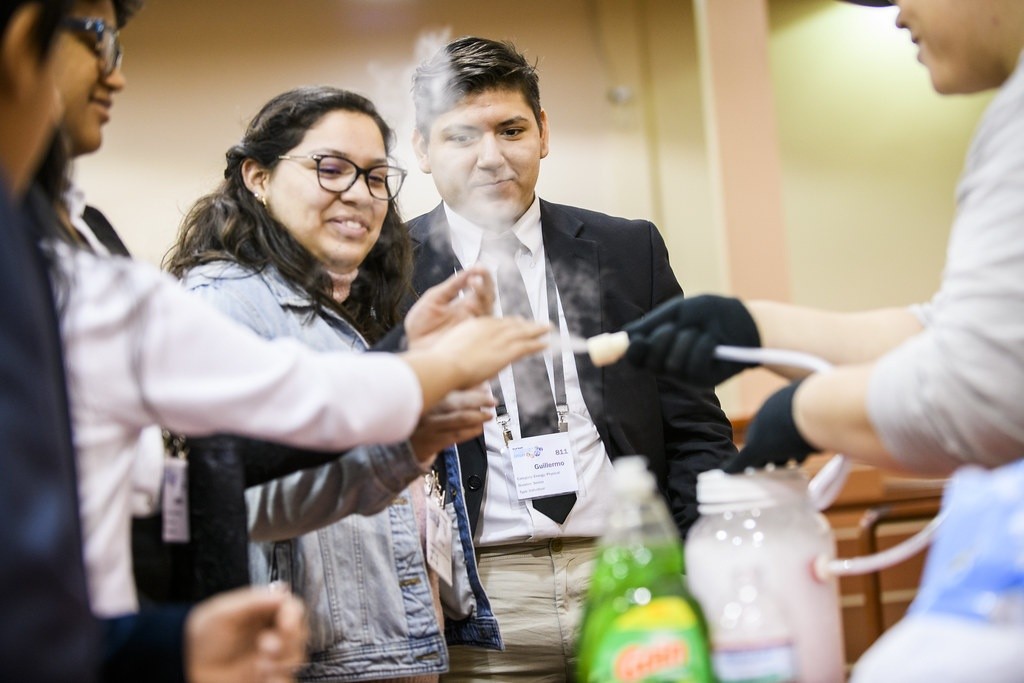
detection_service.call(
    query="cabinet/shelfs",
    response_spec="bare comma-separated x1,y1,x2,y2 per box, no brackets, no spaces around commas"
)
804,453,945,683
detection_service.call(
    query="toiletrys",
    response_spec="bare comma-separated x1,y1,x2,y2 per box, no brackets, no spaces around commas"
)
572,454,715,683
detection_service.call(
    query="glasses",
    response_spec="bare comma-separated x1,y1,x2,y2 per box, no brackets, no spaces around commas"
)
274,154,407,200
56,18,124,74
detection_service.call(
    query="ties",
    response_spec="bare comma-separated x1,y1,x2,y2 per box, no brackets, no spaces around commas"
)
481,235,577,524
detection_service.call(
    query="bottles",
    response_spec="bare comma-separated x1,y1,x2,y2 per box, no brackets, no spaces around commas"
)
684,466,847,683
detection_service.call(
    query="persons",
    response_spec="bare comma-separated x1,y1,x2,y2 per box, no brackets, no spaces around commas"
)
35,0,496,613
625,0,1024,479
0,0,306,683
399,36,739,683
18,126,554,618
160,85,504,683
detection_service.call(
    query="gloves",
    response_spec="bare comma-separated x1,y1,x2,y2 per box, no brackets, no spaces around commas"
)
719,378,822,475
622,294,762,386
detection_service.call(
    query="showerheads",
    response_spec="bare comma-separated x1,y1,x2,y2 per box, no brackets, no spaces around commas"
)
583,329,632,369
